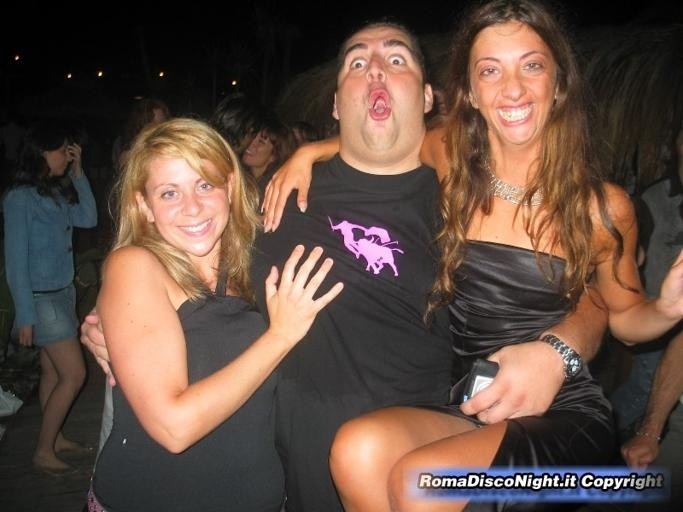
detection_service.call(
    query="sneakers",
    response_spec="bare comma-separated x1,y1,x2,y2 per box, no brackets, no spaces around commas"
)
0,384,24,418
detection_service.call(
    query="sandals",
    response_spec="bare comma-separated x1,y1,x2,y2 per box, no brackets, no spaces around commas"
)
32,445,95,477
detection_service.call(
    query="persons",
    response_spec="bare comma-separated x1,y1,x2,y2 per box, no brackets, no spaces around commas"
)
0,0,683,512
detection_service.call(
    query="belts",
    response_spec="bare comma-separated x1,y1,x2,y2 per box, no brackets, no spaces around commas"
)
31,282,72,295
